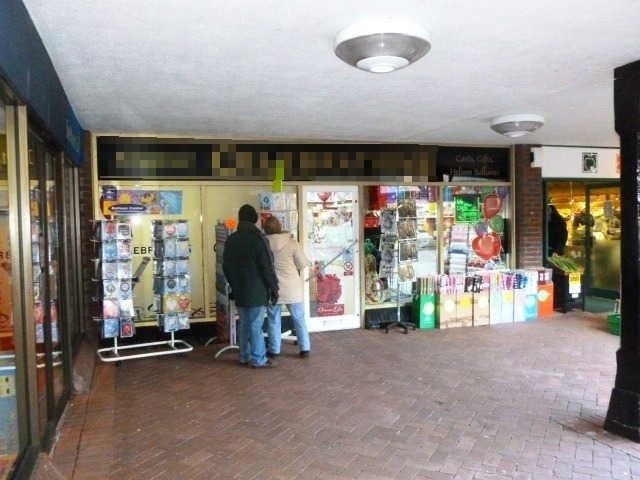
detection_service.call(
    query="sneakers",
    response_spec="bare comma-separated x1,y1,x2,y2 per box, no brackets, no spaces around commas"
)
251,361,272,369
240,359,251,365
266,351,281,358
299,350,311,360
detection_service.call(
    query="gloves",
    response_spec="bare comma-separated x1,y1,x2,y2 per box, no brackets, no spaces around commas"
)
268,289,280,306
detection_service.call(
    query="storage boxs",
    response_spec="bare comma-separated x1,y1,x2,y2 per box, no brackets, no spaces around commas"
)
411,284,554,330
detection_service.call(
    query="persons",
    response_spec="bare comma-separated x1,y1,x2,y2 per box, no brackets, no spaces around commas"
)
260,216,314,357
220,205,280,368
547,205,568,308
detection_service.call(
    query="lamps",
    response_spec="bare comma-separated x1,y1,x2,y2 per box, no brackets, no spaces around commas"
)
491,114,544,138
334,17,432,73
